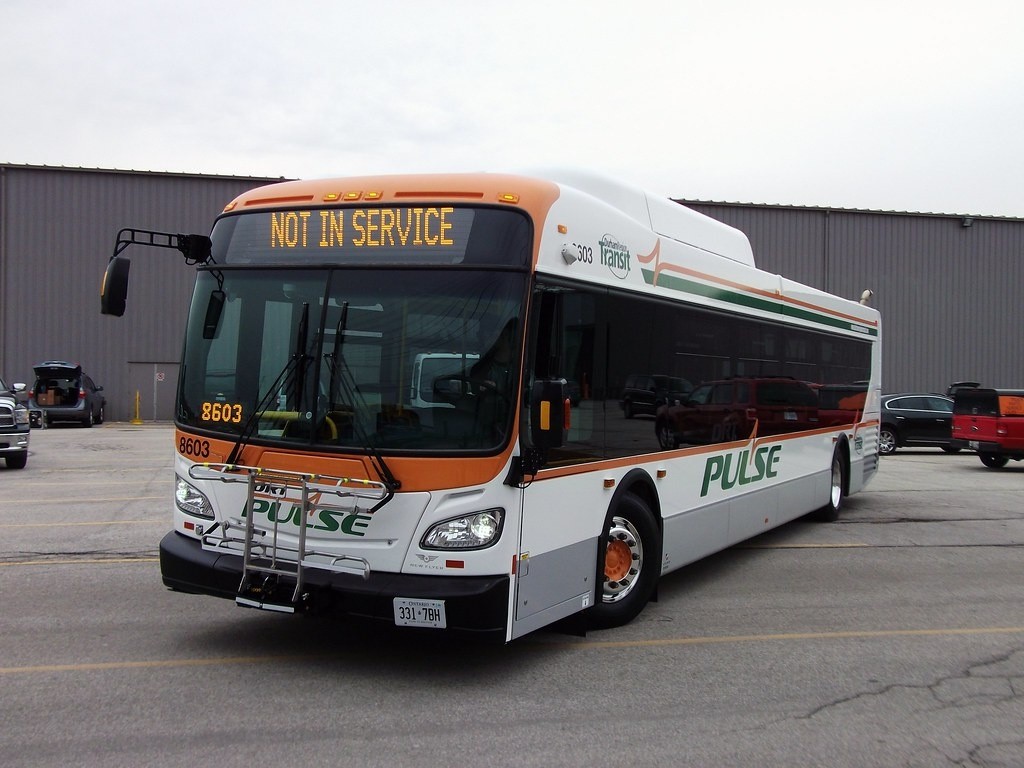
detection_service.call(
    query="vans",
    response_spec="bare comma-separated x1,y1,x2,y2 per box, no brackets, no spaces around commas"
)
276,379,326,415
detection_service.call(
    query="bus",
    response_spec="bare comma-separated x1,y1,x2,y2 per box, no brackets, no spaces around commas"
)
99,158,882,646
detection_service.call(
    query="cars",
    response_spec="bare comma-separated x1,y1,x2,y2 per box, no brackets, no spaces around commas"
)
0,376,30,470
26,360,106,428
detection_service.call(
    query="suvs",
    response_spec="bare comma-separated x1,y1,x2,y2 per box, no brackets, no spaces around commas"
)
951,385,1024,467
619,372,695,419
876,391,955,456
654,374,869,457
